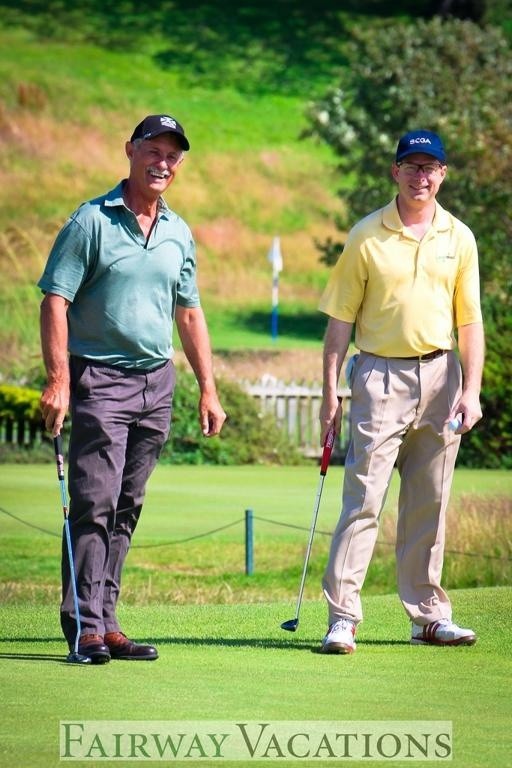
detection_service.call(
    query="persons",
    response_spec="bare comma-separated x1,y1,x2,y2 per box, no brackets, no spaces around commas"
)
316,131,486,656
38,118,229,664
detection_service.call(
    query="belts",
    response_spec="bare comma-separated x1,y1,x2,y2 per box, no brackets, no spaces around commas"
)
403,349,442,360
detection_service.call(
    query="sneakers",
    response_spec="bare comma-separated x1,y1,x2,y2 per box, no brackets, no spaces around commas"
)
411,619,476,644
322,620,356,654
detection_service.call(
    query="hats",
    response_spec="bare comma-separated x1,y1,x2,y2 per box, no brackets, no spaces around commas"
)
131,115,189,151
397,131,446,161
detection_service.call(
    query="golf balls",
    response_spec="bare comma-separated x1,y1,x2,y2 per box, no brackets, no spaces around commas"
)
448,419,460,430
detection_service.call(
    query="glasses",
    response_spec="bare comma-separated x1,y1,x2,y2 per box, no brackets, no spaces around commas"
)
396,161,441,175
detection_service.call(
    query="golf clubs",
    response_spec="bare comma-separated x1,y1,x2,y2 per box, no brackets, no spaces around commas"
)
51,435,91,664
281,420,335,633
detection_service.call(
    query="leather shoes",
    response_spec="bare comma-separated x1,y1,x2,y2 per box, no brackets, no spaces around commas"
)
104,633,158,660
69,634,111,664
